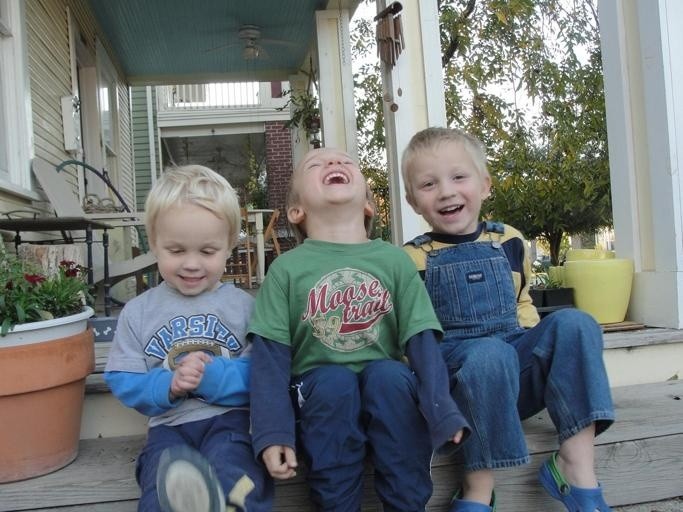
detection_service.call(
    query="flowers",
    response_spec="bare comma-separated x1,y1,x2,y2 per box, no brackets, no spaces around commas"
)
0,235,95,338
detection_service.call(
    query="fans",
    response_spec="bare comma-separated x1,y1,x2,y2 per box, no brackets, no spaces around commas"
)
206,24,298,62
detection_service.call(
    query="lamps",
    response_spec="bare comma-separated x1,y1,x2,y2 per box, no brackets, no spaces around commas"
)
243,39,259,61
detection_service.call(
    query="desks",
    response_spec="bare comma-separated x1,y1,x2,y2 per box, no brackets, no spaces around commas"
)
0,217,115,317
241,209,274,283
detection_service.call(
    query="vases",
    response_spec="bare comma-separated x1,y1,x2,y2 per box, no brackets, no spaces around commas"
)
0,327,96,485
549,266,565,287
564,260,634,324
566,248,615,260
529,290,544,319
0,305,93,349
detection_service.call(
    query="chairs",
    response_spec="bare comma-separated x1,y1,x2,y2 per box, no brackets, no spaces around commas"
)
220,206,253,288
252,209,281,274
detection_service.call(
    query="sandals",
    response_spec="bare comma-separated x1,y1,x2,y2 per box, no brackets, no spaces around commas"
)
448,486,496,511
539,451,611,511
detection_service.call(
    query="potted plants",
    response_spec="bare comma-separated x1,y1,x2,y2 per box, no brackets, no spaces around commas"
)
541,274,574,315
274,89,320,143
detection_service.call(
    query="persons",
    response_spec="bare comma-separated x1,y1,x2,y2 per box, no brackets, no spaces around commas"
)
400,126,616,512
245,148,474,512
104,164,274,512
